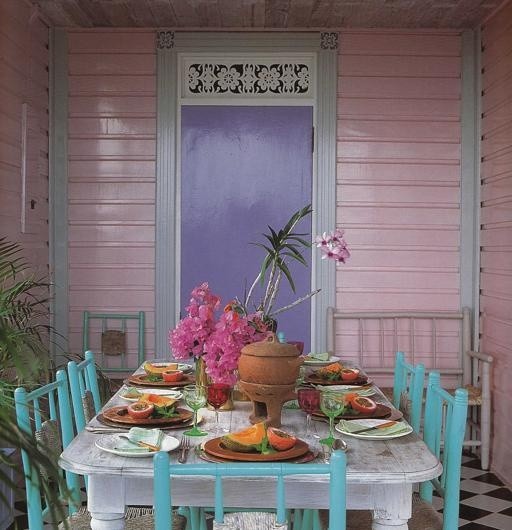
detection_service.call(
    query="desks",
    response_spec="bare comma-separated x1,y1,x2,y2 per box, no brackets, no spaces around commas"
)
55,352,444,529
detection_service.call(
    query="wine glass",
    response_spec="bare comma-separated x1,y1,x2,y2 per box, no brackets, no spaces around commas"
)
184,385,209,438
298,389,318,440
206,381,231,435
319,393,346,447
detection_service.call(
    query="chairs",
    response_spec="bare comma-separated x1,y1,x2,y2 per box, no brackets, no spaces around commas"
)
14,351,189,529
83,310,144,372
154,449,347,529
319,352,470,530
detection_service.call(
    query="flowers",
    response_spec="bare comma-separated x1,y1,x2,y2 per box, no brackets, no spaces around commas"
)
168,205,350,385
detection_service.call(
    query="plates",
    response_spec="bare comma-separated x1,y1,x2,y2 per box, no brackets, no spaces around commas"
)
123,373,195,389
304,371,375,384
197,435,320,463
343,395,404,418
318,384,378,395
300,352,343,366
94,431,177,460
143,360,193,372
337,418,417,441
99,403,205,429
118,387,184,403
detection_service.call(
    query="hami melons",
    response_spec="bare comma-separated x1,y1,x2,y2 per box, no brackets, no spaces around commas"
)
140,394,179,411
220,422,267,453
144,361,178,378
317,361,342,379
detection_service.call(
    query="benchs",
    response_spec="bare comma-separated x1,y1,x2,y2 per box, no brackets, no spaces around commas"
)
325,306,493,470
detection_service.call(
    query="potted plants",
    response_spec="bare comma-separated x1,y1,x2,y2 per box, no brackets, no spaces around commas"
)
0,237,96,530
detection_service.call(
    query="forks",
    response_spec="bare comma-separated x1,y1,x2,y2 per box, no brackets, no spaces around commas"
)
179,436,190,464
